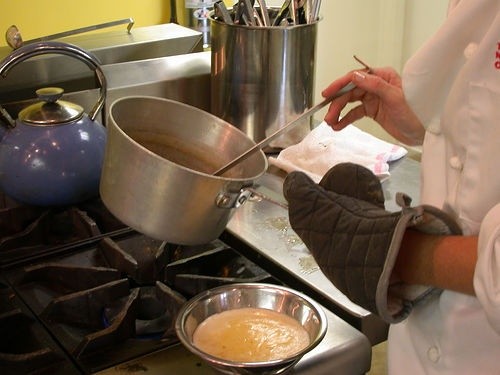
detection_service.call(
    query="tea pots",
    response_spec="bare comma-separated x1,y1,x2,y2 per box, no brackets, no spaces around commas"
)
0,42,108,214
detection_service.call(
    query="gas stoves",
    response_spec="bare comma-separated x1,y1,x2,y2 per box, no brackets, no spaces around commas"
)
0,202,371,375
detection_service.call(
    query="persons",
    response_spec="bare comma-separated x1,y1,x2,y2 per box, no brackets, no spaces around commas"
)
284,0,500,375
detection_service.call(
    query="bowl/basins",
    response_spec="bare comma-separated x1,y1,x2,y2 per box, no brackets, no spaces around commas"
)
175,283,329,374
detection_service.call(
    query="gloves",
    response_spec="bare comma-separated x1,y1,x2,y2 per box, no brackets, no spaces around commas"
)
282,162,465,324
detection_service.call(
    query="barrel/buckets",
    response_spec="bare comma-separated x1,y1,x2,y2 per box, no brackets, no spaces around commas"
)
207,7,324,154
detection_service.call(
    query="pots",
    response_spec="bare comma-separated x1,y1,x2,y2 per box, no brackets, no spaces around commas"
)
99,97,268,246
